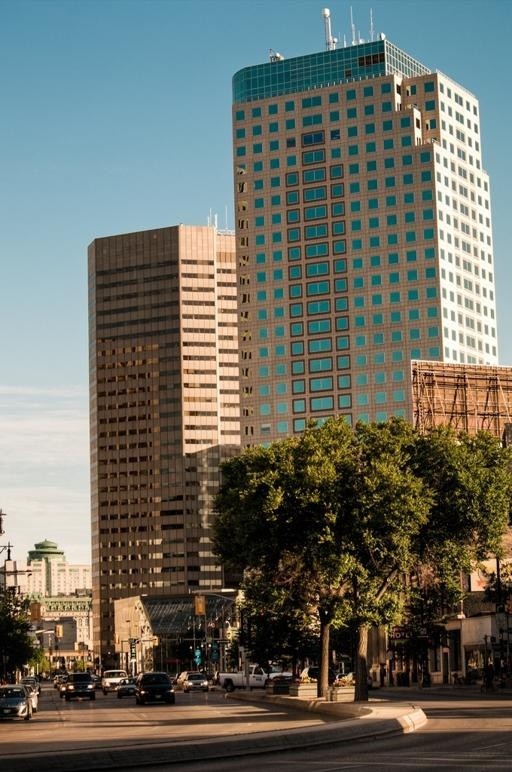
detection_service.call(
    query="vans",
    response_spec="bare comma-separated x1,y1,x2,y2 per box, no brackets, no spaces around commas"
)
102,670,129,697
176,671,199,690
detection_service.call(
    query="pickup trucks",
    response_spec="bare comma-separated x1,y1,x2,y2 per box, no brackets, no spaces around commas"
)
214,660,296,693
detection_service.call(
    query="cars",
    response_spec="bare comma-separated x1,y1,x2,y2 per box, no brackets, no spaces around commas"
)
20,672,102,702
0,684,33,723
115,678,137,699
182,674,210,694
23,684,39,712
297,660,373,690
135,672,177,706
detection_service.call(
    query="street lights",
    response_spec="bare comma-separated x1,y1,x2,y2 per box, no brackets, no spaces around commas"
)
483,633,497,671
186,605,231,671
